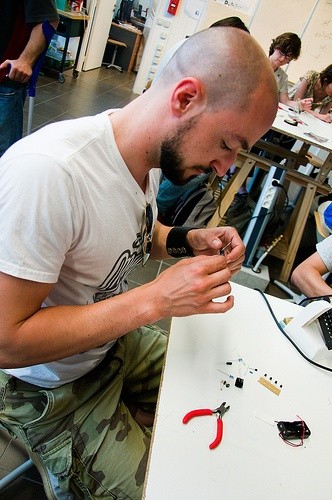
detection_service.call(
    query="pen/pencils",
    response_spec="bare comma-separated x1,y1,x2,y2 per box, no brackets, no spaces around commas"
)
311,103,322,105
328,111,330,113
289,107,297,113
286,114,310,126
278,106,290,113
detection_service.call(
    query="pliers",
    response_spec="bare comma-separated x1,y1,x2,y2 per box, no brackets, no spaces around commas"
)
181,401,231,450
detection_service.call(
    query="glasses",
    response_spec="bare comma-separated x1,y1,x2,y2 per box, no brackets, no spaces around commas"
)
280,50,293,60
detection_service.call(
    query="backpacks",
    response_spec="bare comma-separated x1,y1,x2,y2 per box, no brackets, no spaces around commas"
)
170,181,217,230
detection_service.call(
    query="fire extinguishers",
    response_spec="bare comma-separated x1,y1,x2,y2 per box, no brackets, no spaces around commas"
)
167,0,180,15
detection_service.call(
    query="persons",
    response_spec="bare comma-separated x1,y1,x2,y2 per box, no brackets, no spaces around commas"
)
314,201,332,243
246,63,332,199
151,16,251,223
291,235,332,302
0,27,279,500
0,0,60,159
227,32,313,198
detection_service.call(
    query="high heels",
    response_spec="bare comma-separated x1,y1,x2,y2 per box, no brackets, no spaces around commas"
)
238,192,248,198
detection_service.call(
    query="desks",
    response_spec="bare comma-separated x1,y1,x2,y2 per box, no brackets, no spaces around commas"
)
141,279,331,500
111,22,143,73
40,9,89,83
206,101,332,194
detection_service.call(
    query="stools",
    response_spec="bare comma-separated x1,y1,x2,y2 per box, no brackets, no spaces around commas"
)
102,39,127,73
206,137,332,284
